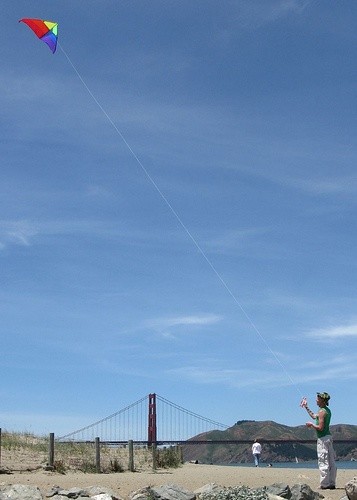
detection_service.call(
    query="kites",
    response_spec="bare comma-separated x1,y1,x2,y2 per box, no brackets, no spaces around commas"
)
19,17,58,53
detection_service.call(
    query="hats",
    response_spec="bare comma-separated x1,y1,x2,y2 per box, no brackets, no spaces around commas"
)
317,392,330,406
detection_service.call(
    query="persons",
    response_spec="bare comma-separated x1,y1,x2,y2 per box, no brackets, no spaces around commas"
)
300,391,337,489
251,438,262,467
